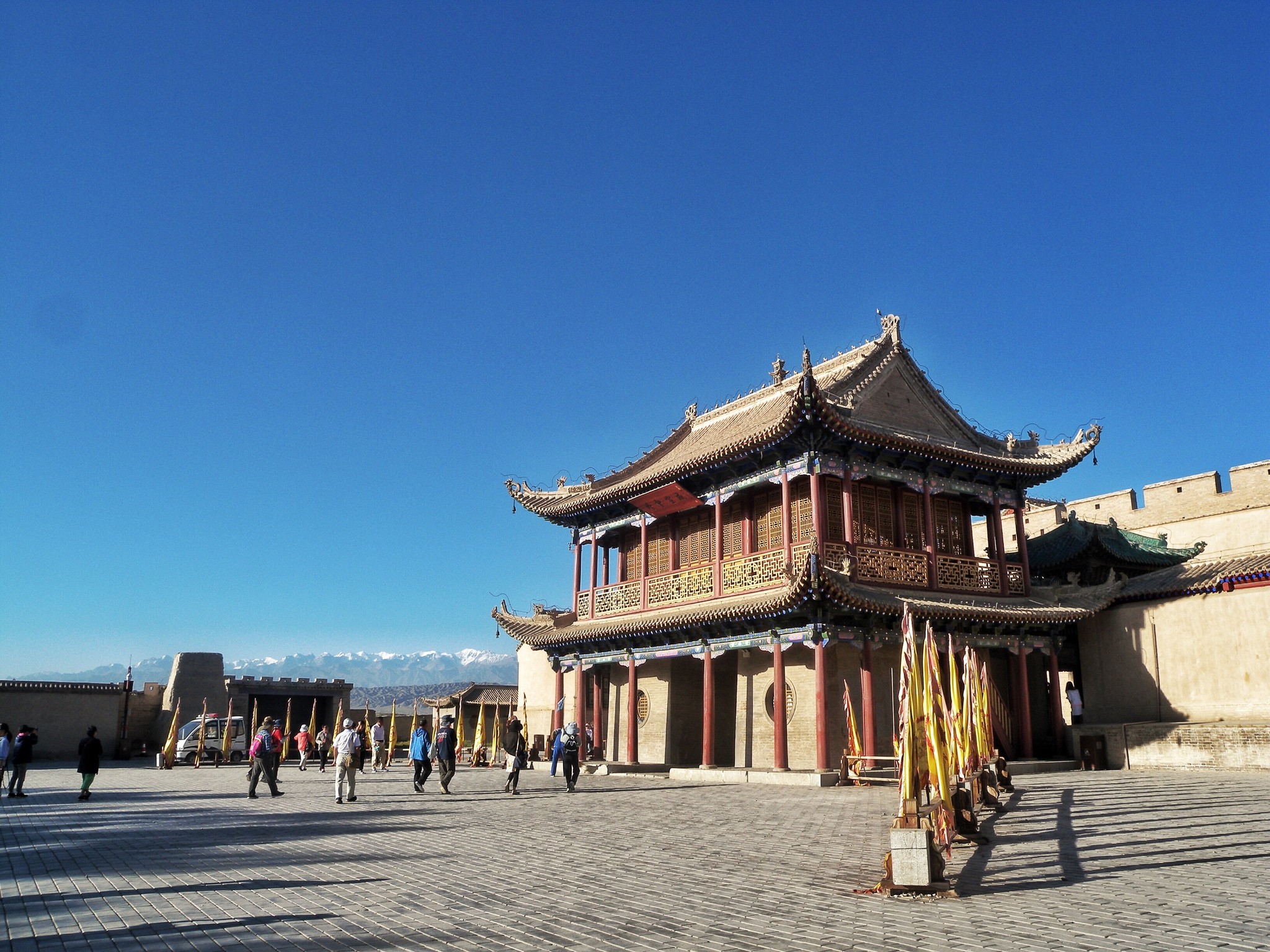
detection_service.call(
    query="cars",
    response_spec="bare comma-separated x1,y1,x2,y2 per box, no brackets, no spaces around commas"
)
176,712,246,764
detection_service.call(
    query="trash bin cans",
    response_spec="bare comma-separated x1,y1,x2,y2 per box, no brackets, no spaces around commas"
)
118,738,131,760
1079,733,1106,772
534,734,545,751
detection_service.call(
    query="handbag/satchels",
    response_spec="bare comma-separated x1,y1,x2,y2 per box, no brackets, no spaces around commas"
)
586,730,591,741
345,754,361,769
427,741,437,760
513,752,528,769
306,741,313,749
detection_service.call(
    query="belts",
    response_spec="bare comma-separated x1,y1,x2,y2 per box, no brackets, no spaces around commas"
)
338,752,349,755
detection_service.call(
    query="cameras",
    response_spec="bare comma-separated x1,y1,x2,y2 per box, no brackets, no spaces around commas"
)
28,726,38,734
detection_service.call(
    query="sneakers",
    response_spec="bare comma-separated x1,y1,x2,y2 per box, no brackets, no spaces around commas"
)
246,774,285,799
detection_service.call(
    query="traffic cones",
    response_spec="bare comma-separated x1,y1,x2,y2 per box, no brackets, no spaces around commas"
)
141,741,146,753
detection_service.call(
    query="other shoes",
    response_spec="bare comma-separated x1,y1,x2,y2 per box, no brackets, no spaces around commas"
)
7,792,29,797
346,795,357,802
567,781,575,793
505,785,510,792
414,781,425,793
438,781,451,794
511,790,520,795
298,765,388,774
78,791,93,802
336,797,342,804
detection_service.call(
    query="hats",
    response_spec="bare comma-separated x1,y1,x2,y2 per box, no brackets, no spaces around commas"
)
299,724,309,733
507,720,523,732
261,716,284,731
343,718,353,728
565,721,580,735
441,715,455,726
585,723,589,726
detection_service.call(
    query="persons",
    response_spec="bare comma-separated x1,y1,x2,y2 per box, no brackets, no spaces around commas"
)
502,720,526,795
370,716,389,773
0,723,13,799
77,725,103,802
315,723,333,773
246,718,285,799
407,719,433,793
207,725,217,737
246,716,290,783
7,724,39,798
434,715,458,795
294,724,314,771
549,720,582,793
333,718,361,804
585,723,593,755
336,720,367,774
1065,681,1084,725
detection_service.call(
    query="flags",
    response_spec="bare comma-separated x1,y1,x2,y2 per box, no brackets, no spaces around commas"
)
194,703,205,768
456,697,529,767
556,697,564,712
279,702,291,763
332,701,440,767
249,700,257,761
221,701,233,763
843,604,995,860
307,701,315,750
163,703,180,769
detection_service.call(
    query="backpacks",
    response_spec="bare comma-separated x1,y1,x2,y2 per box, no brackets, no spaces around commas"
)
564,733,579,754
249,732,273,757
